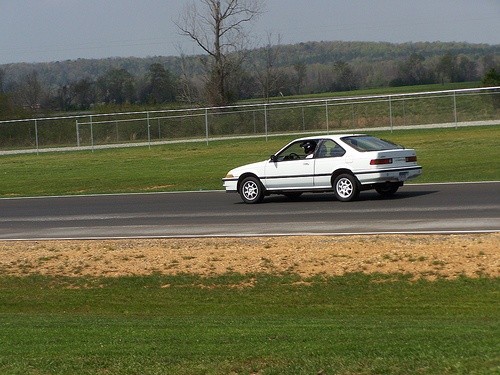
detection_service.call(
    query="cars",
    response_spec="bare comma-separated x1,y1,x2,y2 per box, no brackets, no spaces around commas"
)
222,130,423,201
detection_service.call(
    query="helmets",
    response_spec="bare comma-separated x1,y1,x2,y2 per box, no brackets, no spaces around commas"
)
299,140,318,155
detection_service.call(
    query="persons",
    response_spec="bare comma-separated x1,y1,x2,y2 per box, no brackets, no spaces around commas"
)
301,141,316,159
